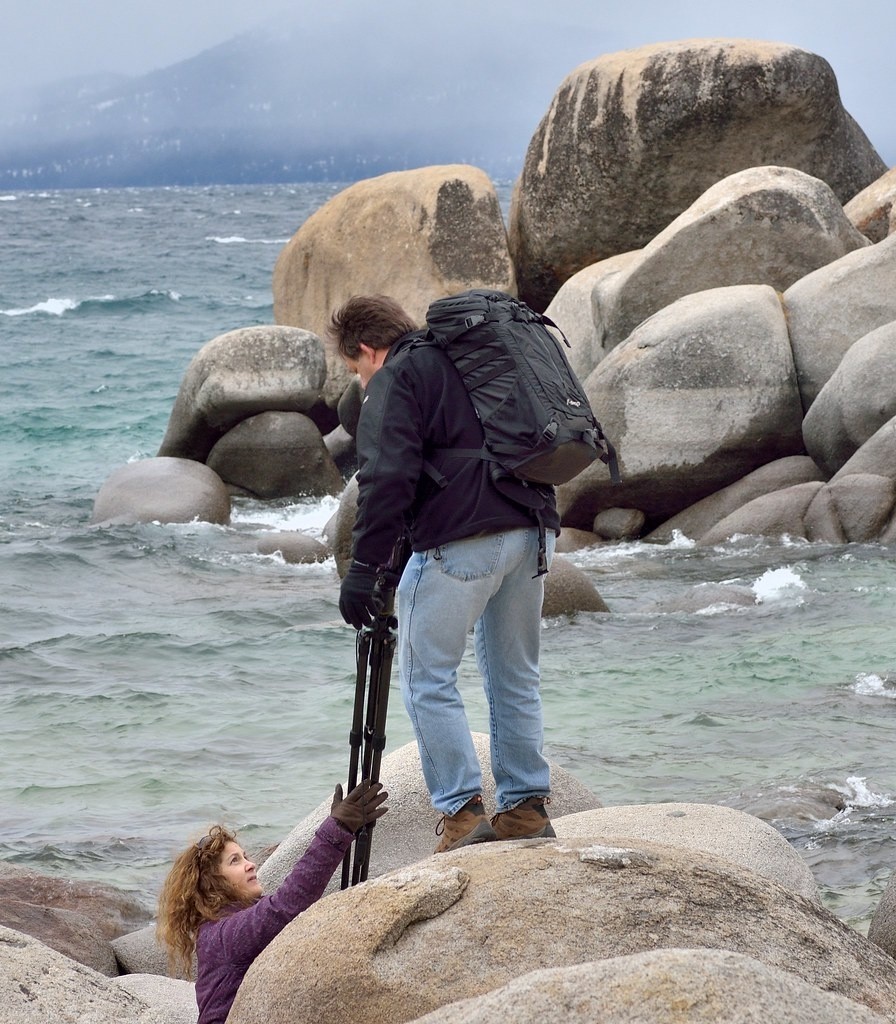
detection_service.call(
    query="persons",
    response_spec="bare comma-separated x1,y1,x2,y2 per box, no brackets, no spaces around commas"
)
327,293,561,855
153,779,388,1024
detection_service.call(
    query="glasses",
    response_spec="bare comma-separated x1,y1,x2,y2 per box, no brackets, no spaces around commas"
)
198,826,221,881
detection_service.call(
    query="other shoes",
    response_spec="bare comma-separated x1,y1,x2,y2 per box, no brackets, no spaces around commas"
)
434,794,496,854
489,795,556,840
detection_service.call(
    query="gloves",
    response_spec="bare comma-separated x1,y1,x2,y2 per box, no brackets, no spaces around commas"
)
339,560,378,630
331,779,389,835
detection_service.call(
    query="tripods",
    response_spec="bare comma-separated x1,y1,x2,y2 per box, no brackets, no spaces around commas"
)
342,591,407,887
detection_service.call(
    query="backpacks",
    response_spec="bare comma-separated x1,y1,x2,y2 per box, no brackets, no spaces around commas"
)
384,289,622,486
